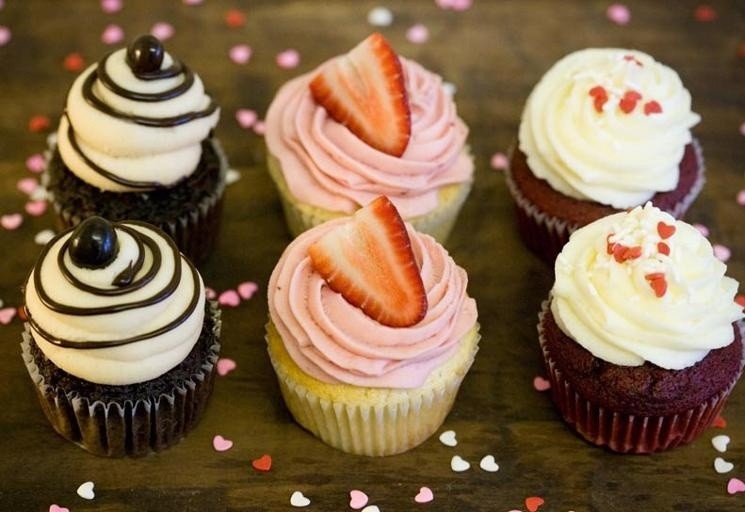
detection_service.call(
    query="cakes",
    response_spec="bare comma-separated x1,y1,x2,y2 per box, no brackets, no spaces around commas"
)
537,200,744,455
44,34,228,269
263,31,476,244
264,195,482,456
19,216,223,459
504,48,706,263
